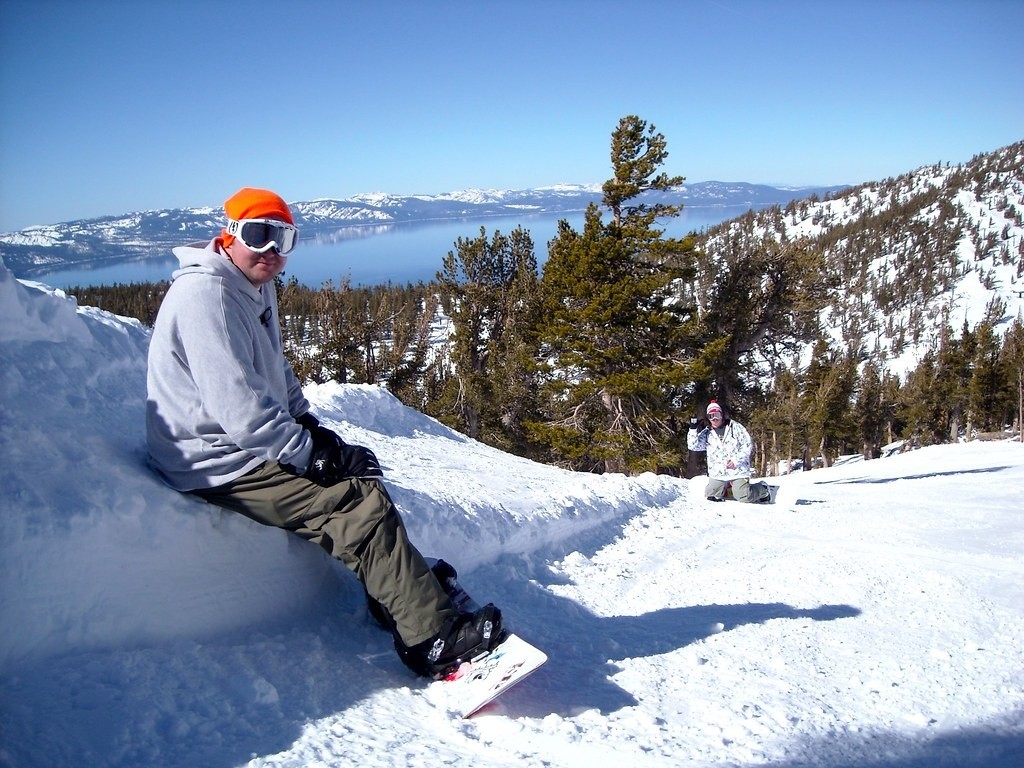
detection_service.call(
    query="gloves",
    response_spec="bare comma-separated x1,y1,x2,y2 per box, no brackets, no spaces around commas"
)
295,411,383,489
688,414,704,429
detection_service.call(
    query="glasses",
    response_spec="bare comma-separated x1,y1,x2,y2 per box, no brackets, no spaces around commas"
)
706,412,722,420
225,215,299,257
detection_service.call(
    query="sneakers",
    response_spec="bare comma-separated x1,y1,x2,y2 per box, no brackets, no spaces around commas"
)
759,480,772,503
370,559,507,681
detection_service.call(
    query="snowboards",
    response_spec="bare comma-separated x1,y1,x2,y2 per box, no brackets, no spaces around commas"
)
721,480,772,505
417,557,549,720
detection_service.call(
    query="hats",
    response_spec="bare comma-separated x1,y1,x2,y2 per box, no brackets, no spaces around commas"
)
707,400,722,415
219,187,294,250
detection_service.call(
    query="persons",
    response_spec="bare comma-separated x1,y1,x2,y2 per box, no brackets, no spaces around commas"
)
686,399,773,504
137,184,505,670
